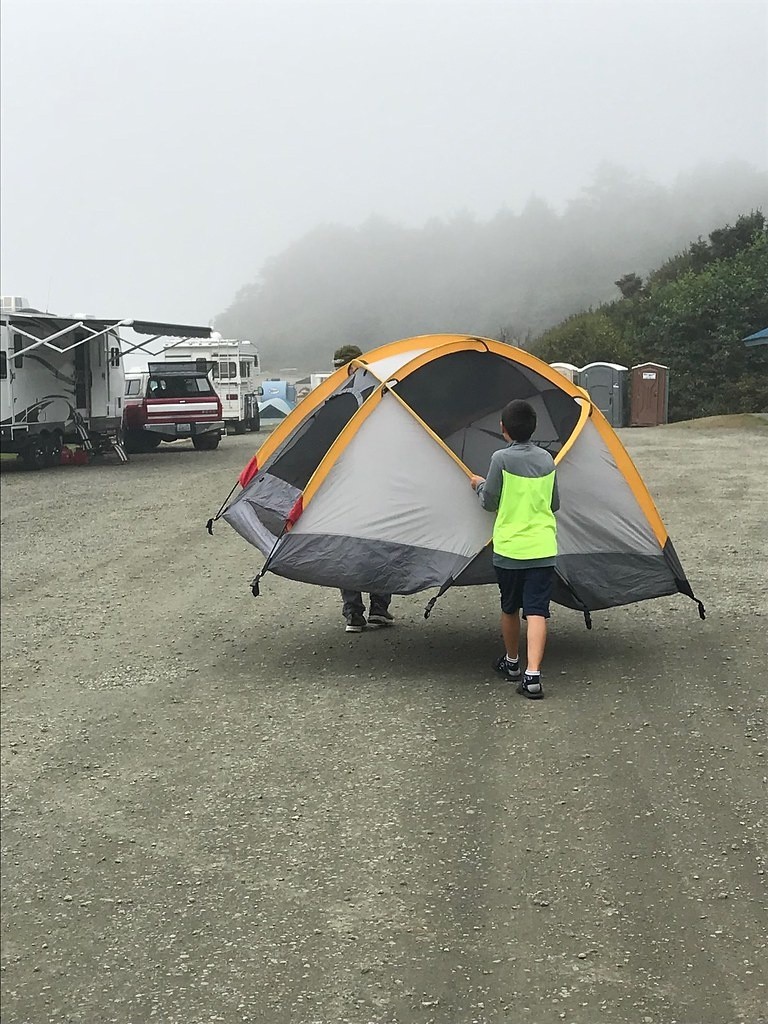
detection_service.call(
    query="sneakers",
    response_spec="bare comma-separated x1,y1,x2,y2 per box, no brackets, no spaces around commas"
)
345,616,367,632
492,654,522,680
367,607,395,624
516,674,544,698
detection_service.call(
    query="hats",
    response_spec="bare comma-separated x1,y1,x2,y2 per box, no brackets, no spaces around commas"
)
333,344,362,367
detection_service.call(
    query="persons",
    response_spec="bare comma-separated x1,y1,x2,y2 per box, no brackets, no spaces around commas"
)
333,345,395,631
470,399,560,699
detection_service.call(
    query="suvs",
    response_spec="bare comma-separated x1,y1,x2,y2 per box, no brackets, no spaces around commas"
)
121,360,225,453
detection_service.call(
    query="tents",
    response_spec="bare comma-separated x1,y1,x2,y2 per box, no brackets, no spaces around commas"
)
206,333,706,629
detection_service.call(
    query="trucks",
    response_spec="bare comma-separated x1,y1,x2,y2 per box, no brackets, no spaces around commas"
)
1,313,213,470
161,325,264,436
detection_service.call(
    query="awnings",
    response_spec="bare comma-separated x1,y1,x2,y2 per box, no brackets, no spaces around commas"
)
7,314,211,354
